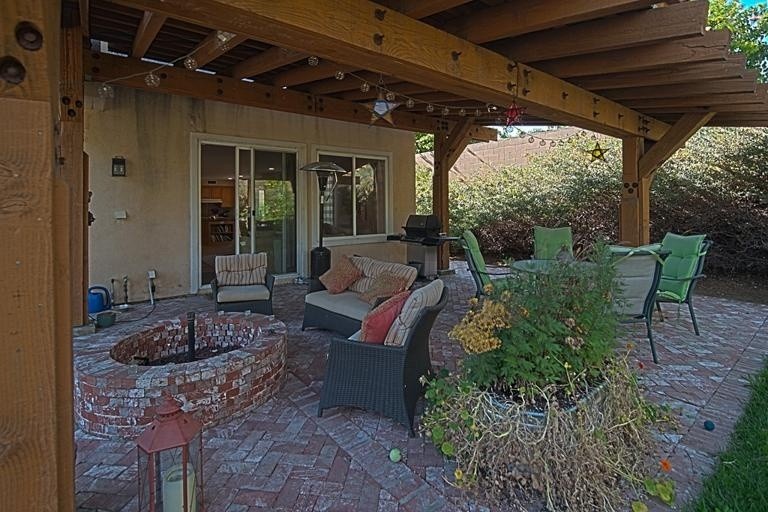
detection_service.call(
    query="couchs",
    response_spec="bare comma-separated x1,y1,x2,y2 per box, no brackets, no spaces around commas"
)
301,249,424,338
210,251,275,317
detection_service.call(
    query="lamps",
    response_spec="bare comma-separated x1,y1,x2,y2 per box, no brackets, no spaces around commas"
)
110,153,123,177
302,159,348,280
135,387,205,512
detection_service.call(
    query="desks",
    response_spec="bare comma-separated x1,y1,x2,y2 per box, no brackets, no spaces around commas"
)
510,258,598,286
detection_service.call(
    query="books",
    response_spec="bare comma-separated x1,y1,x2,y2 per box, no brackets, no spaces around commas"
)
211,223,233,243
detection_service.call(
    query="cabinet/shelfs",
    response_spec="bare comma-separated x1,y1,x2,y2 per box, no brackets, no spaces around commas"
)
202,205,236,247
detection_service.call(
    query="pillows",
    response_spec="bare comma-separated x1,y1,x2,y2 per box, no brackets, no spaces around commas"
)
360,270,406,306
360,290,412,345
315,278,450,439
318,253,363,296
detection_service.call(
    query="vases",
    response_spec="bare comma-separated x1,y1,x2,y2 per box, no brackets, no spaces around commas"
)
427,360,615,494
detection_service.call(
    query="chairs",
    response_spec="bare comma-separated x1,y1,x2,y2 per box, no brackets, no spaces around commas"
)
590,241,671,364
455,228,520,303
530,226,574,262
651,230,713,338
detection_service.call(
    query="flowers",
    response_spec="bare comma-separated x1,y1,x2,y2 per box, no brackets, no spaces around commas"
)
419,246,643,393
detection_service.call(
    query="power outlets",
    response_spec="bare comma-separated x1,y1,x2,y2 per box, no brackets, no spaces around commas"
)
146,269,157,280
